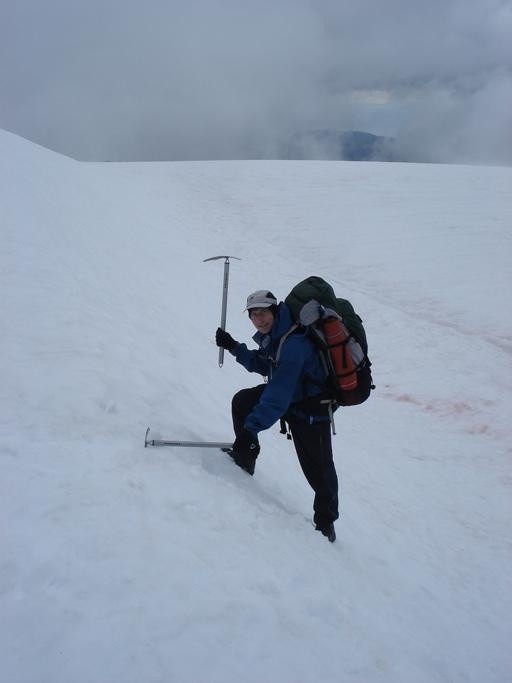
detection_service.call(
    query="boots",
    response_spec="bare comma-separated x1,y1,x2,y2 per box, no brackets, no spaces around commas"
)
221,436,260,477
312,496,339,543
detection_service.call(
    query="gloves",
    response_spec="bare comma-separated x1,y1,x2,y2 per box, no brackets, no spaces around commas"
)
215,327,237,351
232,429,260,459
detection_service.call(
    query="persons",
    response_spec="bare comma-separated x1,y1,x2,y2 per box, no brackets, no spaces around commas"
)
215,288,341,541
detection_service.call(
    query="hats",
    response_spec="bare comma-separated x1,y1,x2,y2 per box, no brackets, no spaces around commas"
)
242,290,277,313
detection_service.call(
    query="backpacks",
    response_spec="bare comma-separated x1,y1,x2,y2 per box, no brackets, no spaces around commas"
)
283,276,376,407
